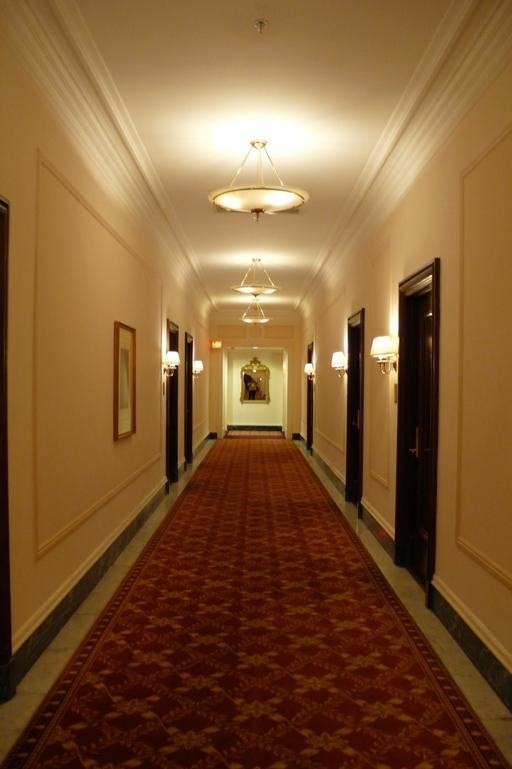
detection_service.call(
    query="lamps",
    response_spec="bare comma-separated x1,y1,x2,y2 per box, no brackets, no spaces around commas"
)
369,336,395,376
331,352,345,378
163,351,181,377
304,363,315,380
192,360,203,380
233,262,277,325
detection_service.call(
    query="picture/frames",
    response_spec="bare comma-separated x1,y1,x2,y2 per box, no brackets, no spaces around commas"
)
242,357,270,403
114,321,136,440
